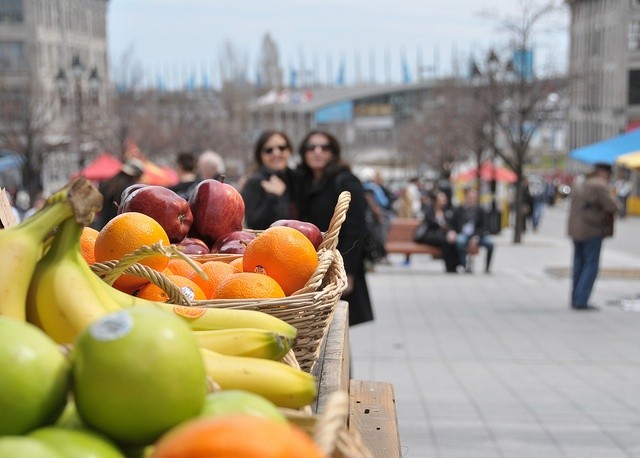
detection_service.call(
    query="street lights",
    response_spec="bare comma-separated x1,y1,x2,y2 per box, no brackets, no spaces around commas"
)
470,53,515,234
55,55,101,169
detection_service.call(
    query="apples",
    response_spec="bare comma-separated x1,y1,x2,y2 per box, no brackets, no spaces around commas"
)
10,317,70,432
216,240,245,257
70,305,209,439
271,219,321,251
179,244,206,255
122,185,193,243
212,230,252,253
188,178,245,237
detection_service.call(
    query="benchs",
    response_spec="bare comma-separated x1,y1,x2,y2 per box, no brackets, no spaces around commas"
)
385,217,479,264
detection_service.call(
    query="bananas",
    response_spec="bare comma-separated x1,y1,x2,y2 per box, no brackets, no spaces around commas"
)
0,175,321,408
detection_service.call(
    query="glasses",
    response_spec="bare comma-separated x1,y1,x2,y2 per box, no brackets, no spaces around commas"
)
305,144,330,151
262,144,289,154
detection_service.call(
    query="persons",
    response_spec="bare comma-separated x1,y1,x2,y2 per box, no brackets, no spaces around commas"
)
520,176,556,231
567,164,618,307
239,130,297,227
166,153,197,196
422,187,460,273
391,174,422,261
453,185,491,269
175,149,226,204
99,157,144,228
293,132,374,327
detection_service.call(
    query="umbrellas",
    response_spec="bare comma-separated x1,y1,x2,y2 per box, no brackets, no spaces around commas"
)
572,127,639,171
455,160,516,196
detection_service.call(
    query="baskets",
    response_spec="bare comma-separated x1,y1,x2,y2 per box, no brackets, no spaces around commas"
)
86,189,352,376
